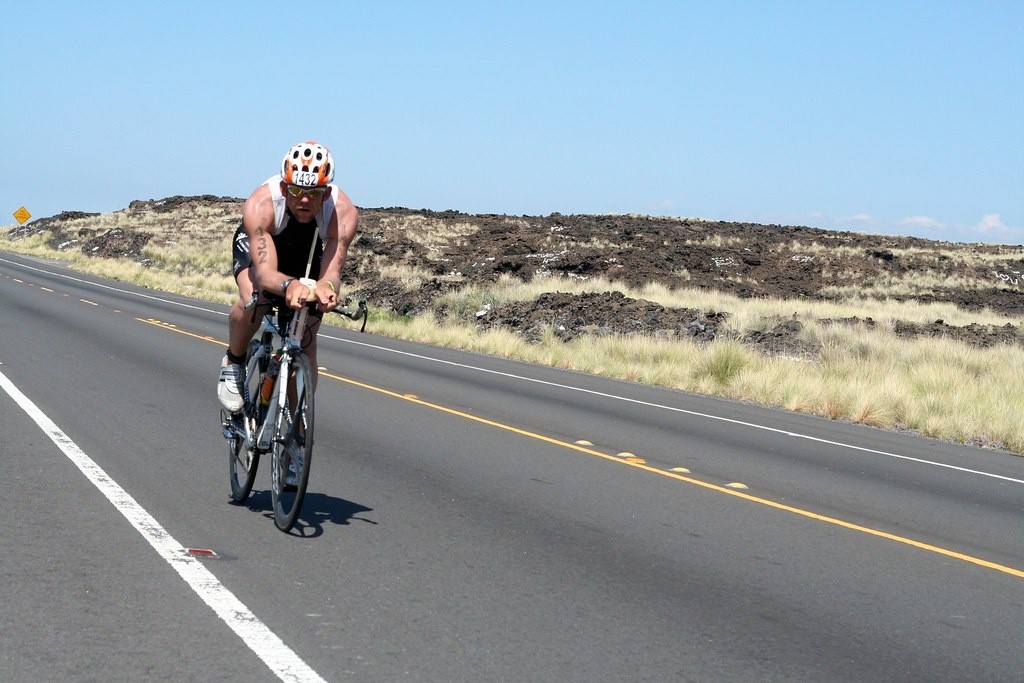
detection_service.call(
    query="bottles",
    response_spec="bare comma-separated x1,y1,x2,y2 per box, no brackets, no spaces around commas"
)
261,349,283,400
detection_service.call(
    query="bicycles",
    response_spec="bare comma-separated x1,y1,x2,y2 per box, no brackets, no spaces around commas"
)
219,225,368,534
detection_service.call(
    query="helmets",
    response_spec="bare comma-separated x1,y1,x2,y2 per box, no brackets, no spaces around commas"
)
281,142,335,187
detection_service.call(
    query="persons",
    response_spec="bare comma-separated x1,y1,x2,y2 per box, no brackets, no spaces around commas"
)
217,141,360,490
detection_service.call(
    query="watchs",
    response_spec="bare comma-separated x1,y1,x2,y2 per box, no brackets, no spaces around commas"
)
281,278,294,292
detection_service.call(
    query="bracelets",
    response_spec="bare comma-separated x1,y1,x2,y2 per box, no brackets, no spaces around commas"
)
327,280,336,292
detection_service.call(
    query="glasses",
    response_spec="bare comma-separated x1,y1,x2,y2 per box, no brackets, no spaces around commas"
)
287,184,326,200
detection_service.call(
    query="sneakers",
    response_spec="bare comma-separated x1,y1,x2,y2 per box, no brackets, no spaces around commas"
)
285,450,305,489
217,349,246,413
